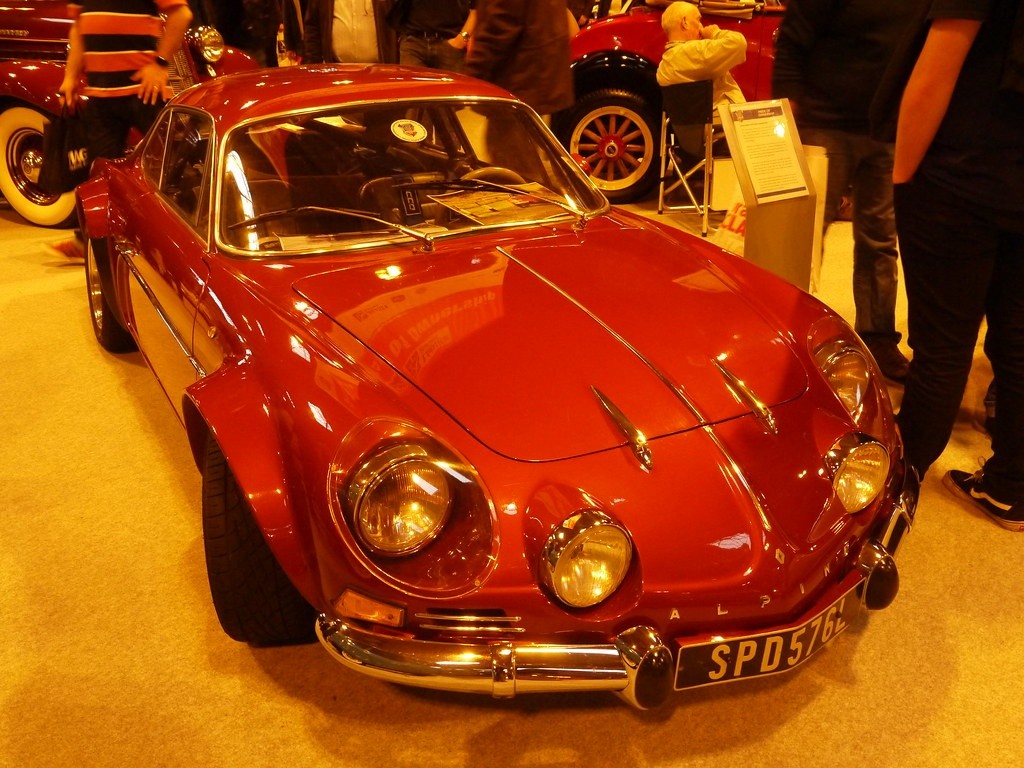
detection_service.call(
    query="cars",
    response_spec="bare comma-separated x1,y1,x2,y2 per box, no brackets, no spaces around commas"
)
0,2,306,227
397,1,977,205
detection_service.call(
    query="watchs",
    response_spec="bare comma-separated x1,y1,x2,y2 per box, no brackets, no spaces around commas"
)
154,56,169,67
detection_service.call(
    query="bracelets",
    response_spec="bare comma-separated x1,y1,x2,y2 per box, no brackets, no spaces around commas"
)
459,32,469,40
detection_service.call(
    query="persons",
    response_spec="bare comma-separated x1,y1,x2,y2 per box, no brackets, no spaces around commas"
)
772,1,911,383
656,2,749,157
567,0,595,28
463,0,579,182
41,0,192,262
383,0,477,74
889,2,1024,531
193,0,391,69
597,0,645,18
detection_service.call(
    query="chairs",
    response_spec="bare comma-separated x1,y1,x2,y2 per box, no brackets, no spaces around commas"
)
358,172,446,232
192,180,303,248
658,79,745,237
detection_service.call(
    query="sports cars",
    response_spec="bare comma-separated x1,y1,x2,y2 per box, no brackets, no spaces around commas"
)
74,65,922,713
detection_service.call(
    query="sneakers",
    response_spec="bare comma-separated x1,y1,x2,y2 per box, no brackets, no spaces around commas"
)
943,456,1024,532
864,331,912,384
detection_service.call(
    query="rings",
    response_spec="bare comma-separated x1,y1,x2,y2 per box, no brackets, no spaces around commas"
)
153,86,158,89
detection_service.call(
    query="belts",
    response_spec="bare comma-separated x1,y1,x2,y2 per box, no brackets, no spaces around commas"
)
406,28,454,40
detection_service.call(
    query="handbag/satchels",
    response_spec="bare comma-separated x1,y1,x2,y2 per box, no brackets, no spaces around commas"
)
36,101,94,195
